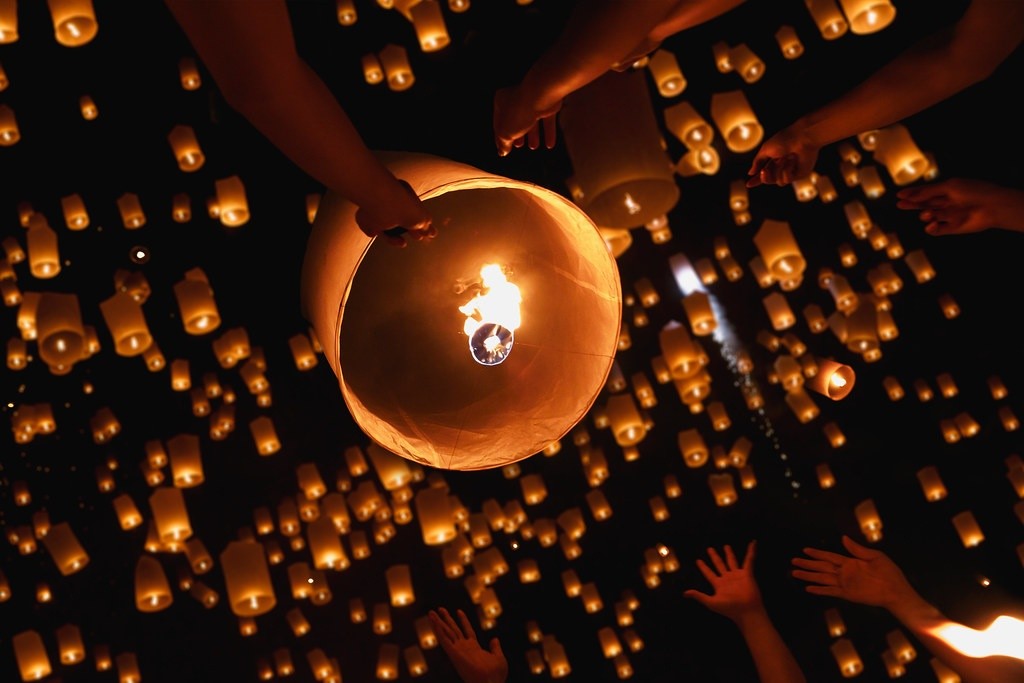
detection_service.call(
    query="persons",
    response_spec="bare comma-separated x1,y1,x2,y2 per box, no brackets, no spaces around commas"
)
427,607,509,683
792,535,1024,683
683,540,806,683
166,0,439,247
896,177,1024,236
493,0,678,157
610,0,746,72
746,0,1024,191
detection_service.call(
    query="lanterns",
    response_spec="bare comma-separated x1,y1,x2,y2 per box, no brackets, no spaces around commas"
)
0,0,1024,683
